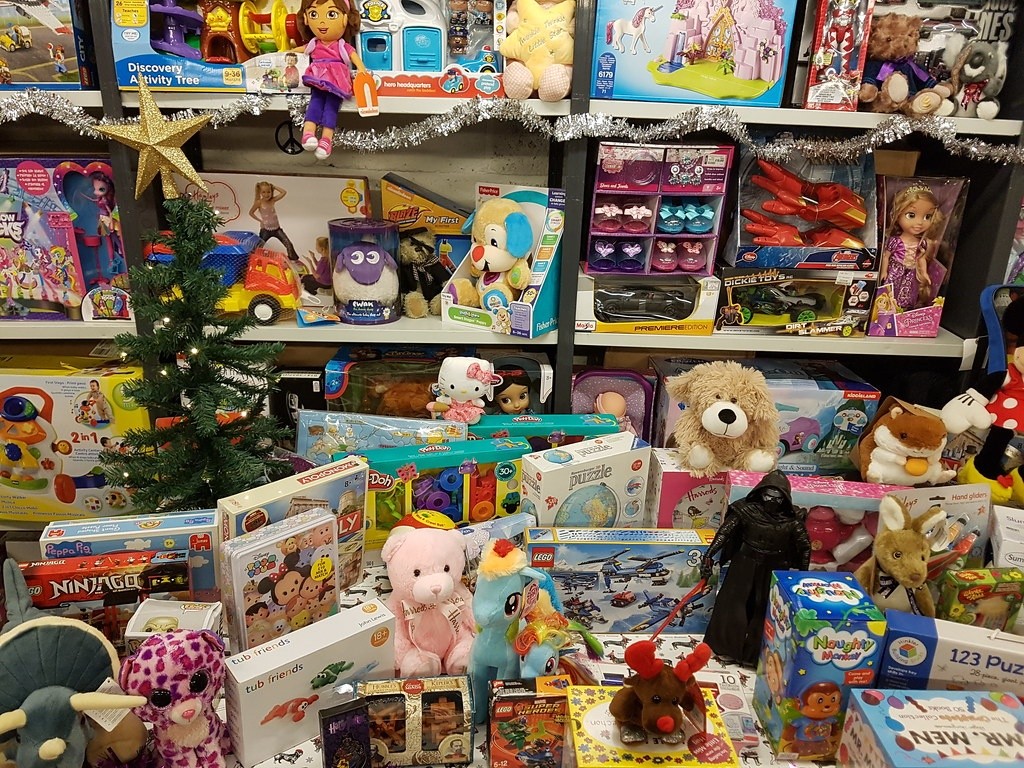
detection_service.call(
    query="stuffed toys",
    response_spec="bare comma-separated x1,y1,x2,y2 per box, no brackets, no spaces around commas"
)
380,524,593,723
854,493,946,616
861,14,1008,119
450,197,531,314
856,406,956,486
665,359,780,479
942,287,1024,506
295,0,366,158
609,639,710,747
426,356,493,425
401,226,448,319
0,558,234,768
502,0,575,103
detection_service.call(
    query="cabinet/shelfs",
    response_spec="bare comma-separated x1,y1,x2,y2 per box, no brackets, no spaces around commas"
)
0,1,1023,561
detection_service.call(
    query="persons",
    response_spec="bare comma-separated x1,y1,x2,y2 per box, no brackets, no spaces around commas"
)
248,181,300,261
300,238,332,294
87,380,115,421
282,52,299,88
